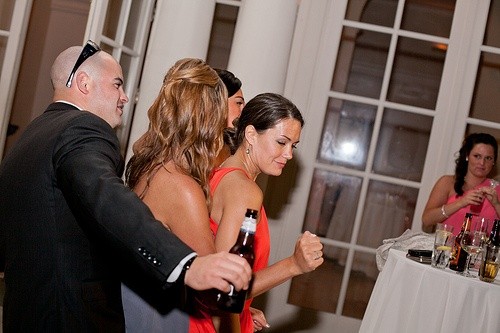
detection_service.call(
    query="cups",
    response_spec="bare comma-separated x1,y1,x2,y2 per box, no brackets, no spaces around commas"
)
431,222,455,264
469,191,487,217
432,229,457,270
479,244,500,283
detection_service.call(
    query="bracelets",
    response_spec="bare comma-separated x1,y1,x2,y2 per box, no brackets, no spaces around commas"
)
441,204,449,218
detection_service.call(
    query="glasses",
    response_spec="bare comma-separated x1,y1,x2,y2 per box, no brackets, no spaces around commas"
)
65,40,102,90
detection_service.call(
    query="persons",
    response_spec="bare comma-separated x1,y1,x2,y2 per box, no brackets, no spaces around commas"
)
209,68,245,169
190,93,305,333
121,58,323,333
422,133,500,239
0,39,252,333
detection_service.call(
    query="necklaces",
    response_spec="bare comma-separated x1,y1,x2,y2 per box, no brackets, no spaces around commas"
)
230,155,252,175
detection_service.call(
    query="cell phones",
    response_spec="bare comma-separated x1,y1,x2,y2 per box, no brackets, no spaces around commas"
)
408,249,432,257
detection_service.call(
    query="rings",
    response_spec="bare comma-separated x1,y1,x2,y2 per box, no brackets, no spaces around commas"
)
314,251,319,259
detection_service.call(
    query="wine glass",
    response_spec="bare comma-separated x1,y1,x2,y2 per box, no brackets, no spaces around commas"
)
456,216,489,278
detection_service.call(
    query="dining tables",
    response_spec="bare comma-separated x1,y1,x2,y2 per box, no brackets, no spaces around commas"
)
357,249,500,333
322,188,407,281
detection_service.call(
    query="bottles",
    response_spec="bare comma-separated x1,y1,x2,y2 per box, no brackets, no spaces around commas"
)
215,207,259,314
449,212,473,272
477,219,500,279
